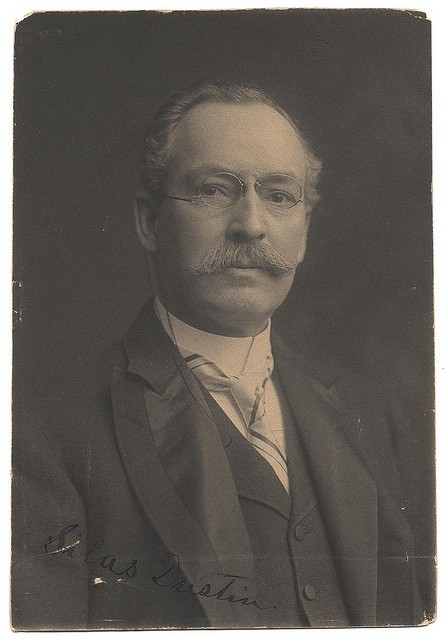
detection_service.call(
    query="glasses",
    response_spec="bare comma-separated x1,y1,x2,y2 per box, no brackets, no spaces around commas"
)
162,172,304,210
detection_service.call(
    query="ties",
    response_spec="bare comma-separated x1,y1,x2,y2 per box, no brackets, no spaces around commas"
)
178,346,289,495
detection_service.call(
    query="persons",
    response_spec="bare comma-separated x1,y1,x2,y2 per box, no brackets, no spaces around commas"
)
12,78,437,631
